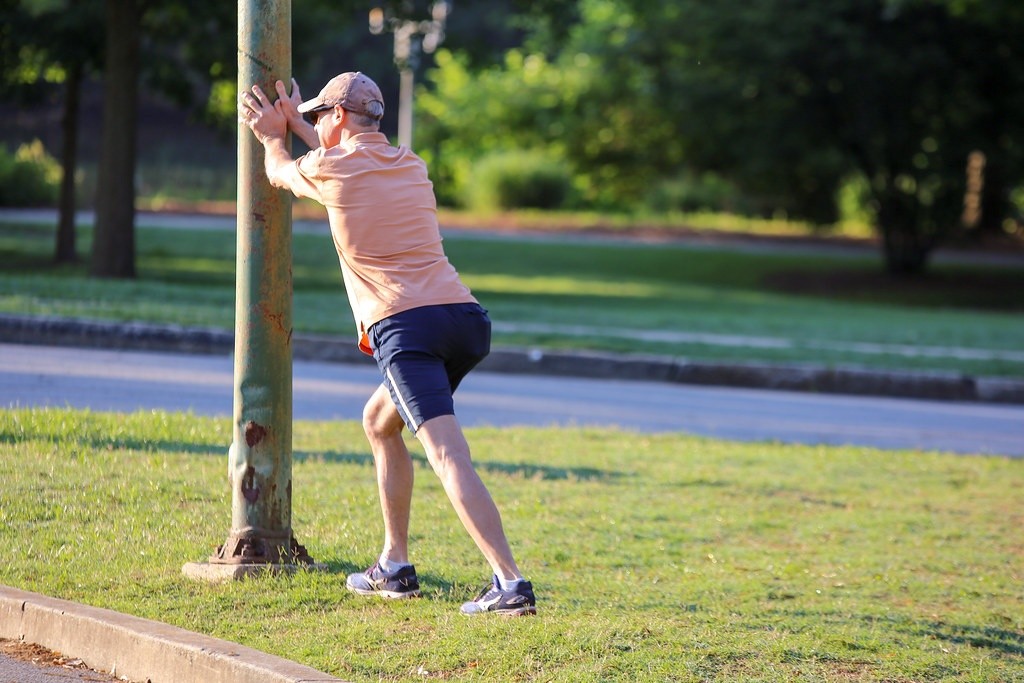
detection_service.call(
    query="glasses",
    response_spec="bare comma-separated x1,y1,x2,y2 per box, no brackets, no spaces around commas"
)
306,107,333,126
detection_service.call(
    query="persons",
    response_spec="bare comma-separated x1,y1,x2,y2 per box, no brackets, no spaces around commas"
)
240,71,539,617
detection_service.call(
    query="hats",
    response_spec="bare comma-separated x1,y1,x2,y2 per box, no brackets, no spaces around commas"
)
297,72,385,121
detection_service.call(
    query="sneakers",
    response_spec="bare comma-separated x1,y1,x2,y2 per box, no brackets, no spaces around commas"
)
347,562,420,598
459,574,537,619
